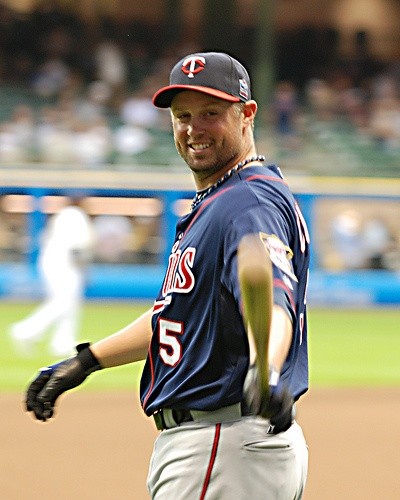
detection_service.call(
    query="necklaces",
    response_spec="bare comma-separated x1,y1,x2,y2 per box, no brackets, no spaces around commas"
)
191,155,265,209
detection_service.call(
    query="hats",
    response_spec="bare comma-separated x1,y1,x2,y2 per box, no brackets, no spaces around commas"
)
153,52,251,109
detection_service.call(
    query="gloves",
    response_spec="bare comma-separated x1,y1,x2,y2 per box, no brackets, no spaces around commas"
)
245,364,294,436
26,342,103,421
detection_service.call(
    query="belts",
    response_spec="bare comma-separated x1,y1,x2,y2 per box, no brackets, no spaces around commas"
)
153,403,251,430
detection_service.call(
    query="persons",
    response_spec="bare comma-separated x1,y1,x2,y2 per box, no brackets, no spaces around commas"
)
11,189,95,357
26,52,309,500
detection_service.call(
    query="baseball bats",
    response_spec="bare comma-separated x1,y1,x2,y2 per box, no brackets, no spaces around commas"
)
239,234,274,402
